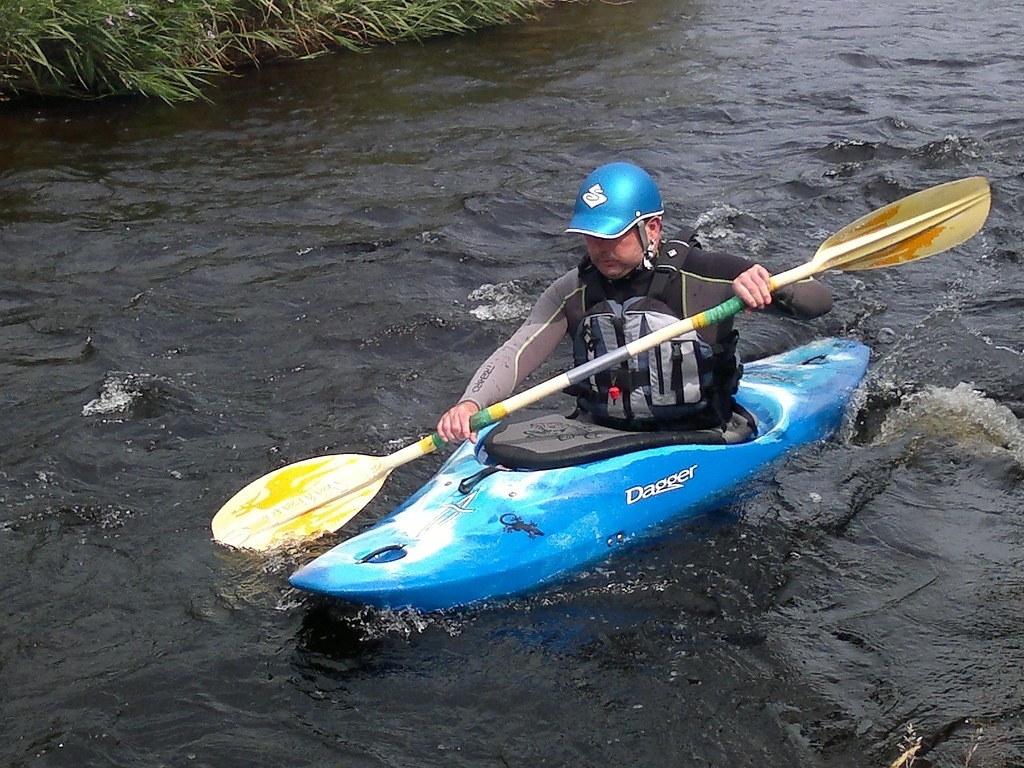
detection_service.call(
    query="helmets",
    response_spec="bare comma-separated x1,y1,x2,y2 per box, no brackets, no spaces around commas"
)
565,162,664,239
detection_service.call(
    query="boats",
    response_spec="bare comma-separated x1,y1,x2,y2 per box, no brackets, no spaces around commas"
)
289,332,876,620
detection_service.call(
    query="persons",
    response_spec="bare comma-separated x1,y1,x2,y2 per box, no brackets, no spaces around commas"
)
435,174,837,464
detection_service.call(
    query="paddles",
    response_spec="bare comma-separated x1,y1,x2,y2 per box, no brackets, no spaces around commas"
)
212,173,992,552
